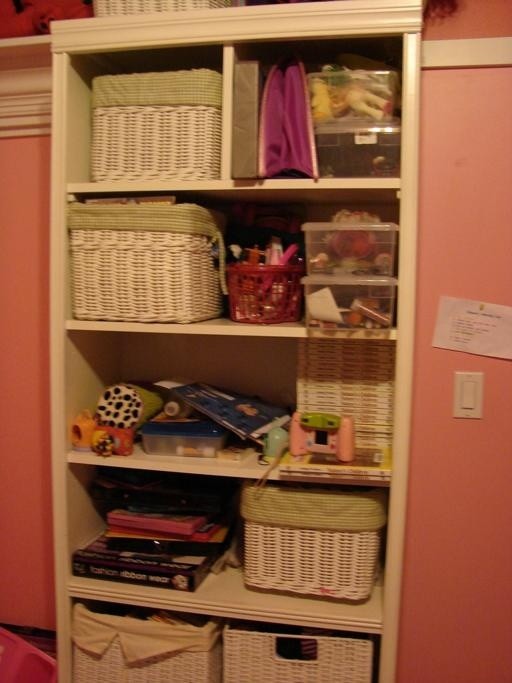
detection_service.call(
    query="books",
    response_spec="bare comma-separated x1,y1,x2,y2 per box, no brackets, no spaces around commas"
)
73,507,231,593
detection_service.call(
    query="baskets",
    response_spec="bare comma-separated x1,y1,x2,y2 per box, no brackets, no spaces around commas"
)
296,338,395,463
93,1,246,17
224,624,373,682
90,69,222,185
71,601,375,683
67,202,306,325
71,603,222,681
238,480,388,602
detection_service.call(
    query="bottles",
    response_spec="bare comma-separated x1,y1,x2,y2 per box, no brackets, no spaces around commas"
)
267,236,290,300
160,397,194,419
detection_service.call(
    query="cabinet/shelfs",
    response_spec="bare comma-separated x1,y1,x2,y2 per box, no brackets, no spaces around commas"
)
50,2,423,682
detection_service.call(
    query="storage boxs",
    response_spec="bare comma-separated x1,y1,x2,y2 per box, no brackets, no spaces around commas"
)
301,276,398,328
137,421,229,458
313,124,401,177
307,71,398,128
302,222,398,275
